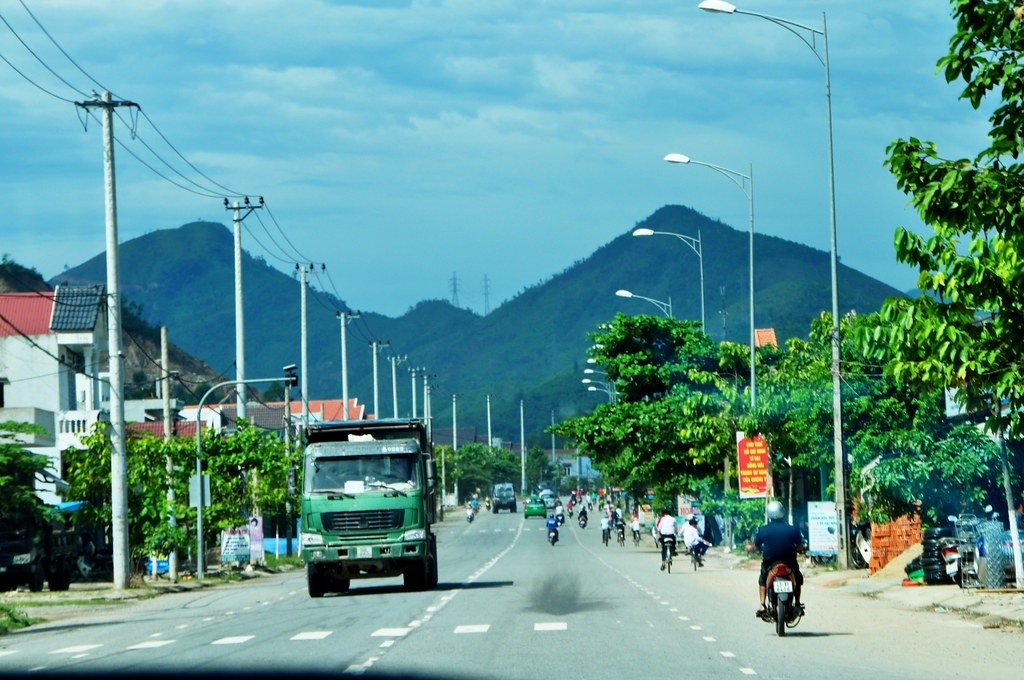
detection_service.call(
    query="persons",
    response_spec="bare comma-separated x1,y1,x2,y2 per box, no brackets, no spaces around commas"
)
250,519,259,530
546,488,597,542
754,501,805,611
598,497,641,543
656,510,713,570
467,493,490,509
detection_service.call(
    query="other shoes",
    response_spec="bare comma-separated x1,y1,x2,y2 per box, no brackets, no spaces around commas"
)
672,552,678,556
756,608,766,617
699,563,703,566
801,608,804,616
661,565,665,570
685,552,689,555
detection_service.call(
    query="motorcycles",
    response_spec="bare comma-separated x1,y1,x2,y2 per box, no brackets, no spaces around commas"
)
746,536,806,638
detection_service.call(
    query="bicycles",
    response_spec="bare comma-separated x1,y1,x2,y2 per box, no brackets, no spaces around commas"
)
656,530,677,574
546,495,640,548
680,536,700,571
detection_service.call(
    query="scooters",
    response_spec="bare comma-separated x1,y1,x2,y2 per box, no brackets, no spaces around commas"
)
465,502,491,524
931,504,994,589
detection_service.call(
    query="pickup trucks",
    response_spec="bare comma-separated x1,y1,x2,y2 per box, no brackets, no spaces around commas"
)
0,500,83,593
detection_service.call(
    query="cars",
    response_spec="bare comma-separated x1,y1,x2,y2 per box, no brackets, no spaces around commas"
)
537,489,559,509
522,495,548,519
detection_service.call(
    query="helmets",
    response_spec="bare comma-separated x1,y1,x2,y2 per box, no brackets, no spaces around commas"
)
767,500,785,520
550,514,554,518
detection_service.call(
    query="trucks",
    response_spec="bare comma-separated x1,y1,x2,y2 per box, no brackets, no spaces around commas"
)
490,483,517,514
287,417,440,597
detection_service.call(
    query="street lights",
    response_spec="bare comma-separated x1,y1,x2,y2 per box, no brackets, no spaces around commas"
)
615,289,675,322
697,0,854,571
632,228,735,553
661,152,760,430
